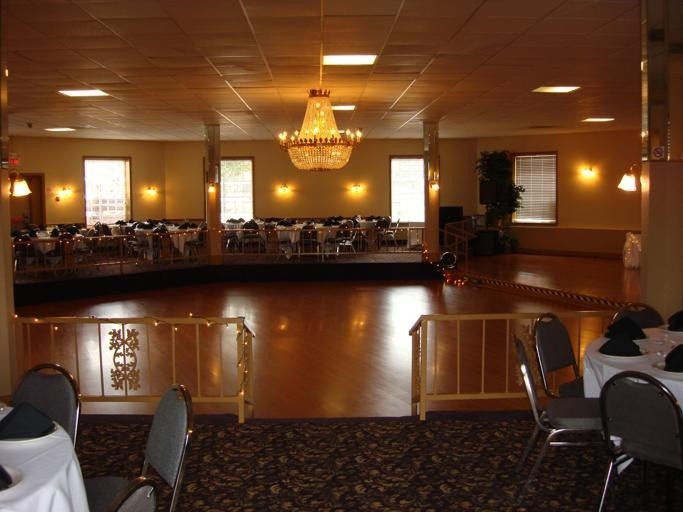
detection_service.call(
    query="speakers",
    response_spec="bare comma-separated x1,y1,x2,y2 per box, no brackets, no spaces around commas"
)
479,177,498,205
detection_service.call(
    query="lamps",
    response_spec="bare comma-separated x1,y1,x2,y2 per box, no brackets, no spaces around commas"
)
278,0,362,171
6,169,32,197
617,159,641,192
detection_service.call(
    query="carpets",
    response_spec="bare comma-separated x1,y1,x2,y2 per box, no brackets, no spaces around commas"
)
78,409,679,510
15,261,450,306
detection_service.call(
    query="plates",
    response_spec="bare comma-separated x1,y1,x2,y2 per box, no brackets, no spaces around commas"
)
662,325,683,334
0,422,59,444
602,332,650,341
596,347,649,358
1,464,25,494
653,359,682,375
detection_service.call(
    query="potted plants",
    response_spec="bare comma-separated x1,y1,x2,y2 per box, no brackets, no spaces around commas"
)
476,151,525,255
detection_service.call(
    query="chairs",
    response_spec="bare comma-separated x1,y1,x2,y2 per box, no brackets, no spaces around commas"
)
512,302,682,511
11,364,193,511
11,214,401,275
